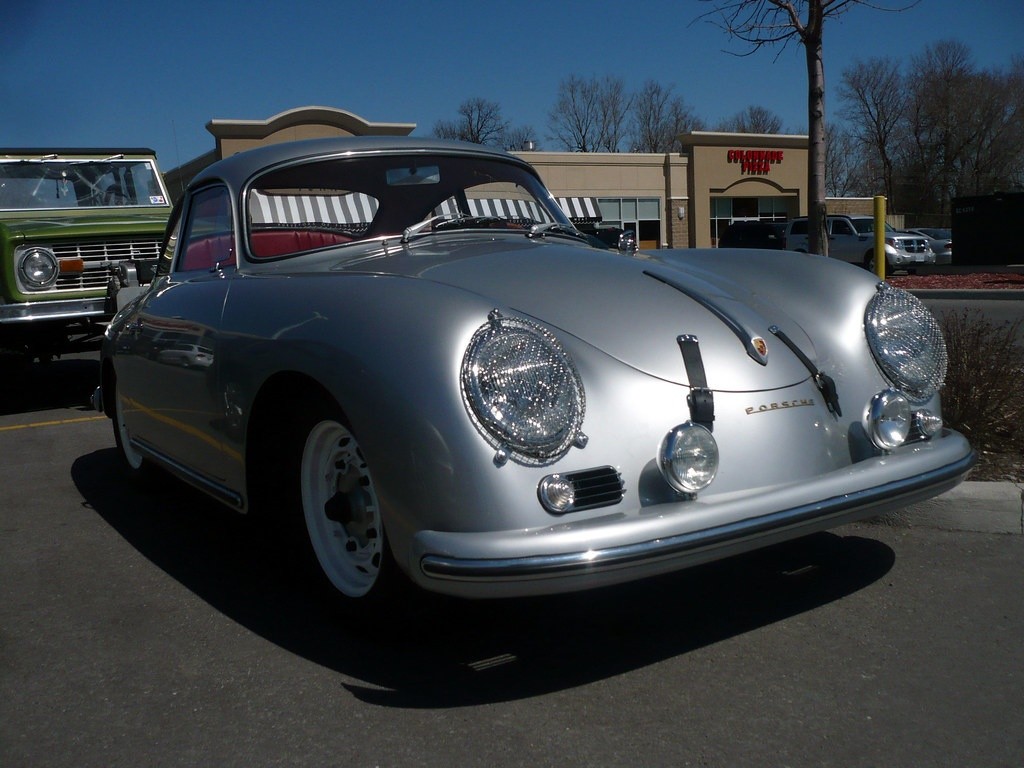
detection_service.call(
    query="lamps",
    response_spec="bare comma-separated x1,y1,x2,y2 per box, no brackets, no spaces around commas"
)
678,207,684,218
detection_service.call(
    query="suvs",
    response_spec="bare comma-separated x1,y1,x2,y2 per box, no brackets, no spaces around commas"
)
718,220,785,249
0,148,230,384
783,213,936,277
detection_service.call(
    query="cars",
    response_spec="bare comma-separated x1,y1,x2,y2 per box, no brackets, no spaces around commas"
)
97,137,974,606
901,227,952,265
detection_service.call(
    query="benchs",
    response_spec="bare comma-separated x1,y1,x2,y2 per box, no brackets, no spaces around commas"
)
183,226,360,270
